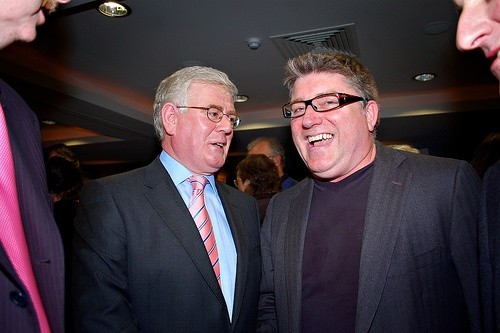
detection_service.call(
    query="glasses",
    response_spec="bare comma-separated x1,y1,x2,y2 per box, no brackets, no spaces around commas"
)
233,179,246,188
281,92,368,118
175,105,242,130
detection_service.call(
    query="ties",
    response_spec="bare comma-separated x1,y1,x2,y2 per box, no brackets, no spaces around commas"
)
185,174,222,289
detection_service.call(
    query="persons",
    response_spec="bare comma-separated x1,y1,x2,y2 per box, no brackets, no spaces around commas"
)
0,0,72,333
50,0,500,333
